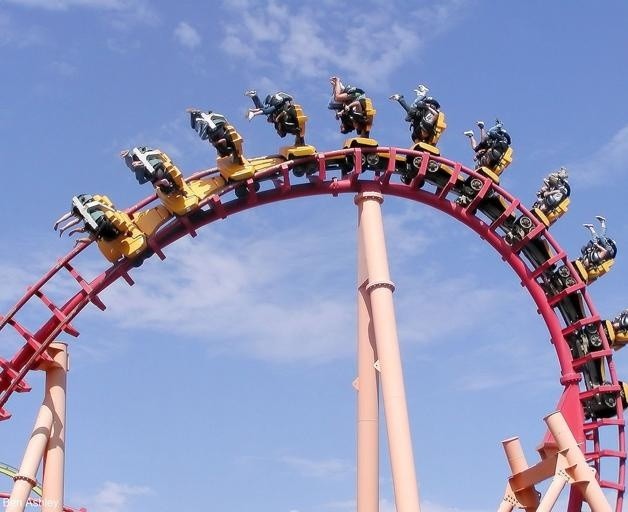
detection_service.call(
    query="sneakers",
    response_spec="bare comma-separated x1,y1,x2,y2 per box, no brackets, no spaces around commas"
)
389,84,429,100
583,216,605,228
244,90,256,122
464,122,484,138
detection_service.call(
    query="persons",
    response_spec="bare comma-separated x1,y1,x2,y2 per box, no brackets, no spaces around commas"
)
482,122,506,165
328,77,364,132
611,309,628,332
582,215,610,269
194,117,229,159
388,92,425,143
410,84,438,139
535,167,570,211
131,158,173,195
244,90,274,123
186,107,203,129
462,121,488,166
52,202,83,238
68,222,98,247
326,80,348,132
119,147,145,172
246,102,289,132
